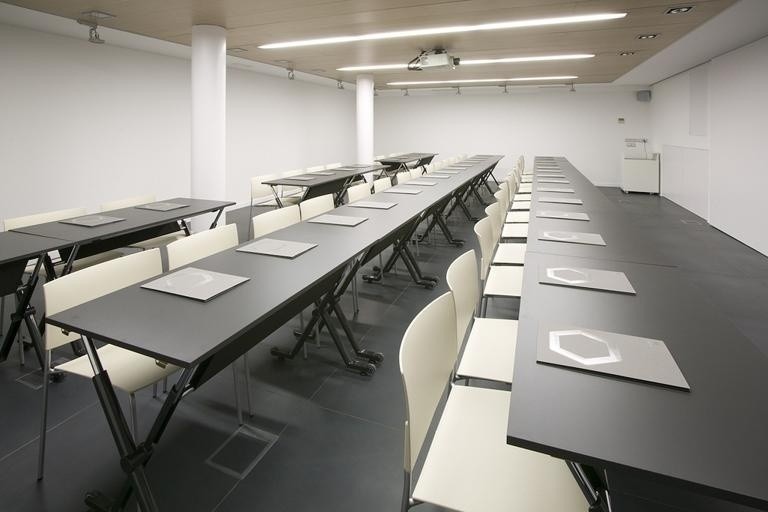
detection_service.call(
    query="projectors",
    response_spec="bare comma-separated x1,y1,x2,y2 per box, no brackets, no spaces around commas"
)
409,55,461,70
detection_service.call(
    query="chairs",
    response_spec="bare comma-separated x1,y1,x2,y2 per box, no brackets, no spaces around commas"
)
37,248,242,481
166,224,307,418
399,291,588,511
250,151,524,365
447,248,519,388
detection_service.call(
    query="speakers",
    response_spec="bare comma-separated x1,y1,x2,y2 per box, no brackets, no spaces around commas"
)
637,90,651,101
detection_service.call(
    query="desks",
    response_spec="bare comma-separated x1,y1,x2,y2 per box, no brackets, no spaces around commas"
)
45,242,377,511
10,197,236,384
506,156,768,511
2,231,76,380
